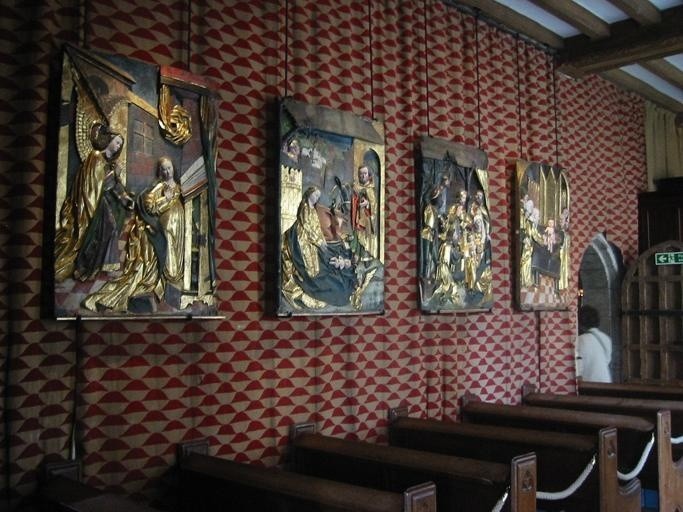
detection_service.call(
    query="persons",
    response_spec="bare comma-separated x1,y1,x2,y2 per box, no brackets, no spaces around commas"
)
80,155,185,317
54,131,135,283
422,175,491,307
520,190,568,292
350,164,376,257
577,304,612,386
281,184,360,311
